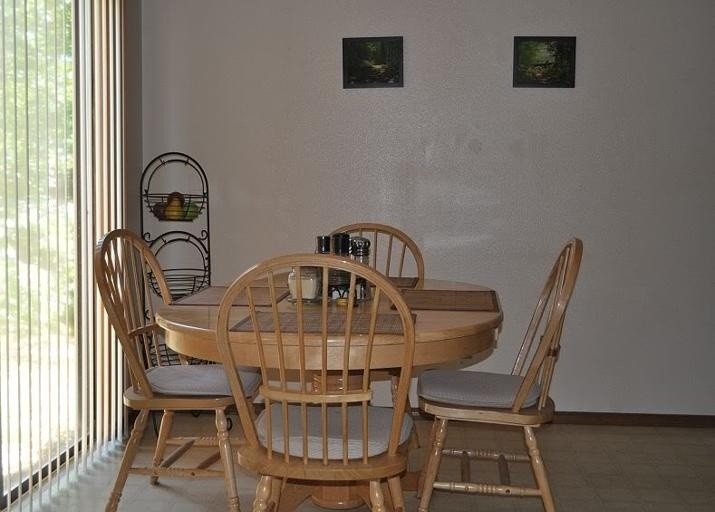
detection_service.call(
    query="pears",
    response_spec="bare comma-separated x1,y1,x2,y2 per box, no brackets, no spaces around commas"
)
164,197,183,220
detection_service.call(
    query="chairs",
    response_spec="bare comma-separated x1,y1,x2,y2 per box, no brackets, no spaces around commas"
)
92,222,584,511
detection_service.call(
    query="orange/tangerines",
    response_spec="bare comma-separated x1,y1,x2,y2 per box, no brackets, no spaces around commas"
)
183,203,198,218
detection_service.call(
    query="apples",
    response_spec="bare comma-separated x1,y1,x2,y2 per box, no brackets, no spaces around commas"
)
166,192,185,206
153,202,168,219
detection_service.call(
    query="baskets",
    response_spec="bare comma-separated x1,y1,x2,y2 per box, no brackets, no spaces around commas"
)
148,344,216,368
148,269,211,303
146,193,207,221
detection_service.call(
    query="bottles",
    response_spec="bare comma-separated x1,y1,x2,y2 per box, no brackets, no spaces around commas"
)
287,265,322,301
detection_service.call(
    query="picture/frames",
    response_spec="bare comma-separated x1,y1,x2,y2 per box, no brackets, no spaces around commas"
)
341,36,405,89
513,37,576,89
